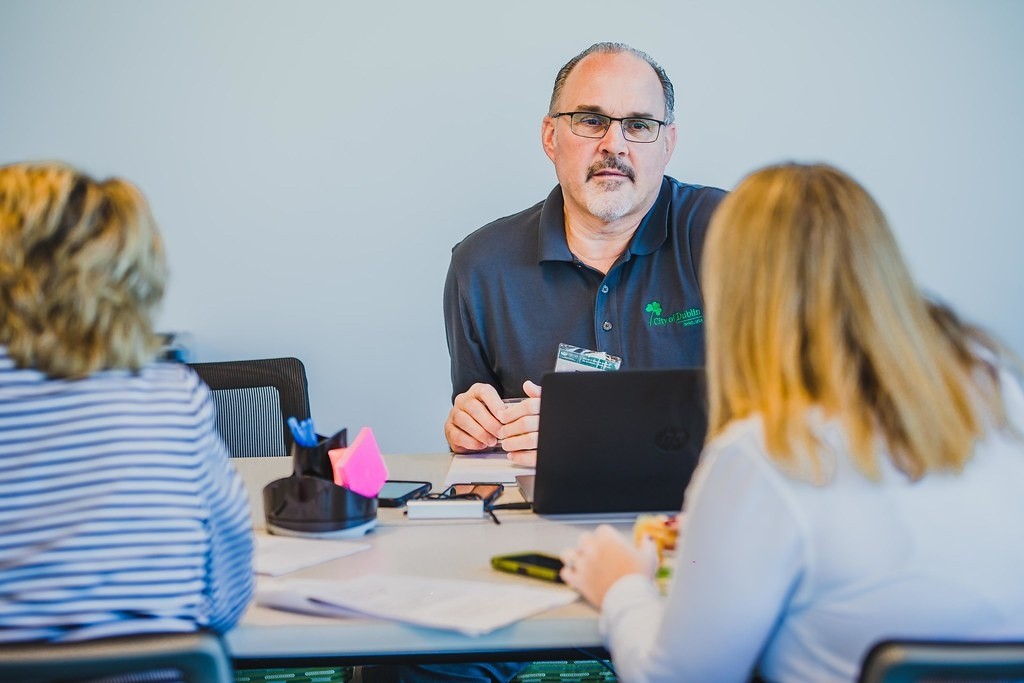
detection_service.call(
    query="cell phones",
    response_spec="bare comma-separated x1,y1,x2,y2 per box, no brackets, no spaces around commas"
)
376,480,432,507
491,550,568,583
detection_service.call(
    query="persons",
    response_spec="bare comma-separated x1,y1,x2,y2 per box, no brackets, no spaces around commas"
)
443,42,732,466
559,163,1024,683
0,161,257,683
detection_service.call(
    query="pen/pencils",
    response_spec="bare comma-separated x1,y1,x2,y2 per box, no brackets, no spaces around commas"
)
283,415,319,450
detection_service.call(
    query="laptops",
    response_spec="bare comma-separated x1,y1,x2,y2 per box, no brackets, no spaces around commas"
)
515,368,707,518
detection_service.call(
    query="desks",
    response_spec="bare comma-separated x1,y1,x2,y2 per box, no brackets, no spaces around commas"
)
221,452,635,669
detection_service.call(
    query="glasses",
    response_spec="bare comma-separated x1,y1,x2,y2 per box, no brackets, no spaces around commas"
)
549,110,666,143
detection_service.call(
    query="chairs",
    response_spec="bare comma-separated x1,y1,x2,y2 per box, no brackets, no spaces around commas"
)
187,357,311,458
